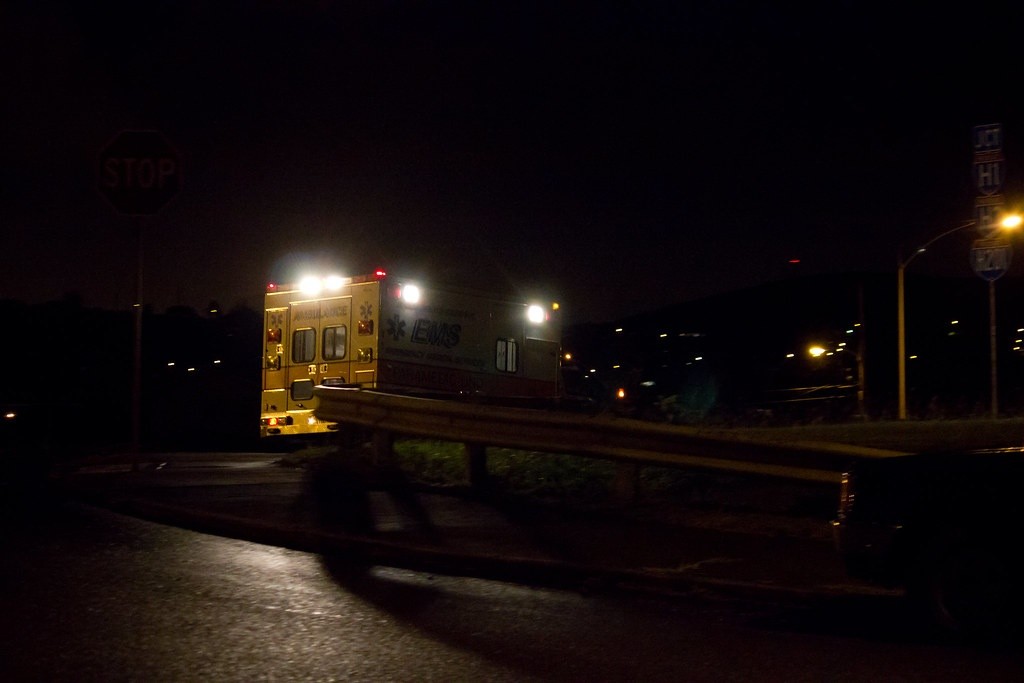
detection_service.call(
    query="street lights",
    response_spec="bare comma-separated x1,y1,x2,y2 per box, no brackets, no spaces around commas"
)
894,205,1022,428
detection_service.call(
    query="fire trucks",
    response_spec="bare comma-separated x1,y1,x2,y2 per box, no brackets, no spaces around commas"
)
259,272,616,451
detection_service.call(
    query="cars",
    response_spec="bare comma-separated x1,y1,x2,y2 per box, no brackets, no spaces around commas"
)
828,447,1024,637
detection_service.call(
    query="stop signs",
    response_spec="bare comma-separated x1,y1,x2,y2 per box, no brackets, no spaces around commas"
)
94,129,186,221
